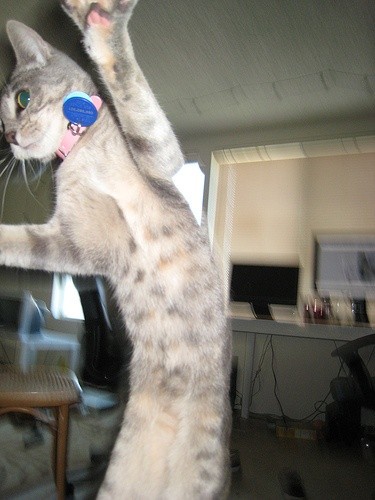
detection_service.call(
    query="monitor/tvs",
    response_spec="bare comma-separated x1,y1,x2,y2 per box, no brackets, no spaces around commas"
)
229,262,299,320
0,294,43,335
312,233,375,325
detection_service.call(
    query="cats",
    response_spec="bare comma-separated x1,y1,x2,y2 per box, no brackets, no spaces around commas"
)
1,0,233,499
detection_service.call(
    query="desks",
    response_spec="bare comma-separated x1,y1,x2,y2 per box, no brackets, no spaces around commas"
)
228,311,375,419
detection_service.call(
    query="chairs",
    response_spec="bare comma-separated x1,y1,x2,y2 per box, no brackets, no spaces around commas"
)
0,362,83,500
